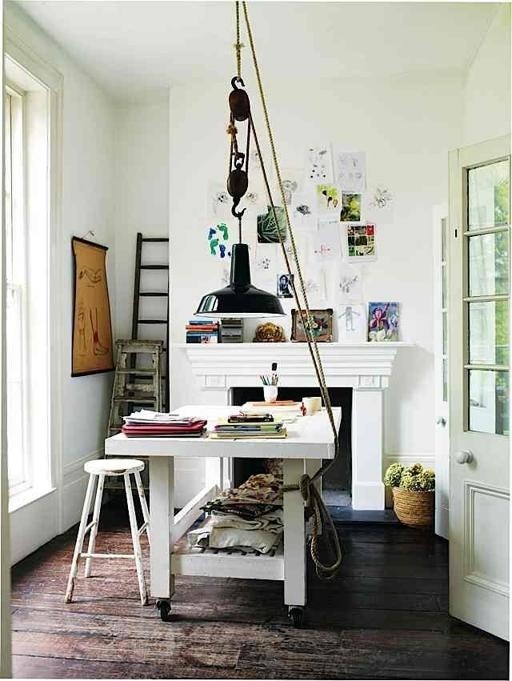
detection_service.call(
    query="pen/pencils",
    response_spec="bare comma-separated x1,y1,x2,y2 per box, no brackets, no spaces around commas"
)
259,373,279,386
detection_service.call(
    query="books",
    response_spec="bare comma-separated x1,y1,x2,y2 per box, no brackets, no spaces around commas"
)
121,409,287,439
185,318,243,343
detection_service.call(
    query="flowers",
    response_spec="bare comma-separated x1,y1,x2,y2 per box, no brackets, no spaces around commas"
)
382,460,434,492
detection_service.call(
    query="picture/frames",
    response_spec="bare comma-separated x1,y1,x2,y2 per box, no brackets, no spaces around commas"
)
289,309,334,343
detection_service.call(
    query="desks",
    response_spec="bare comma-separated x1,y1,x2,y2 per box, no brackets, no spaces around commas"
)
104,405,343,606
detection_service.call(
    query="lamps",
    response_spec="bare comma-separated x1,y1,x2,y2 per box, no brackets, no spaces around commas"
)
192,208,291,325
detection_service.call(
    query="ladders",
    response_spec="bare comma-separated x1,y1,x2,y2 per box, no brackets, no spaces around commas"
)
127,232,169,416
95,338,165,491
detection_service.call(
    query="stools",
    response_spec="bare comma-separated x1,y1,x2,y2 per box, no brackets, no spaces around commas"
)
62,457,153,606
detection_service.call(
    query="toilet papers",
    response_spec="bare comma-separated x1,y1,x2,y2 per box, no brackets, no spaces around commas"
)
302,395,322,417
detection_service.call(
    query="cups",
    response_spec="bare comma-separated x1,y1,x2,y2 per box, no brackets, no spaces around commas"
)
264,385,279,404
303,396,319,416
